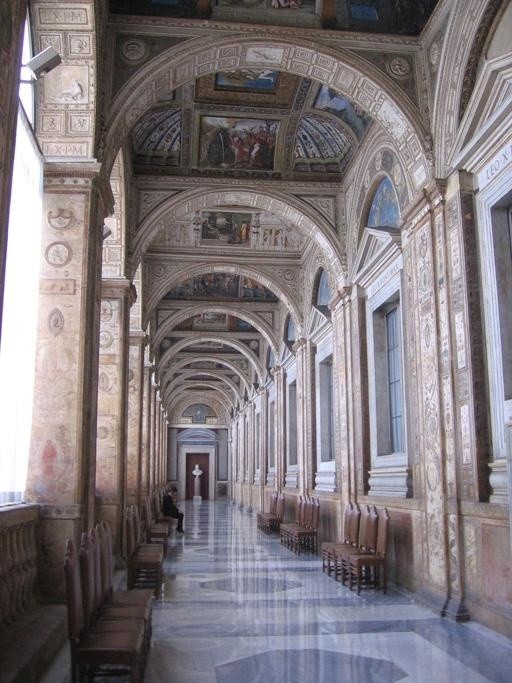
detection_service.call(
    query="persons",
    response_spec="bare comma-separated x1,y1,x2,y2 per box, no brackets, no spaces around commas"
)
192,463,203,476
160,482,186,534
189,124,279,298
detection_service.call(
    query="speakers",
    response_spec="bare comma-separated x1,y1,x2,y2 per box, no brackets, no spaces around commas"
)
28,46,61,80
103,226,111,239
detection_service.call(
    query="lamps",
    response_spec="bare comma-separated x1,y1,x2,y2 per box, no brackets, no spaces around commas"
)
20,45,61,84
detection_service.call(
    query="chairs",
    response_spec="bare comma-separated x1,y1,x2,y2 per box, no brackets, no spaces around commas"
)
62,482,175,682
256,491,392,595
